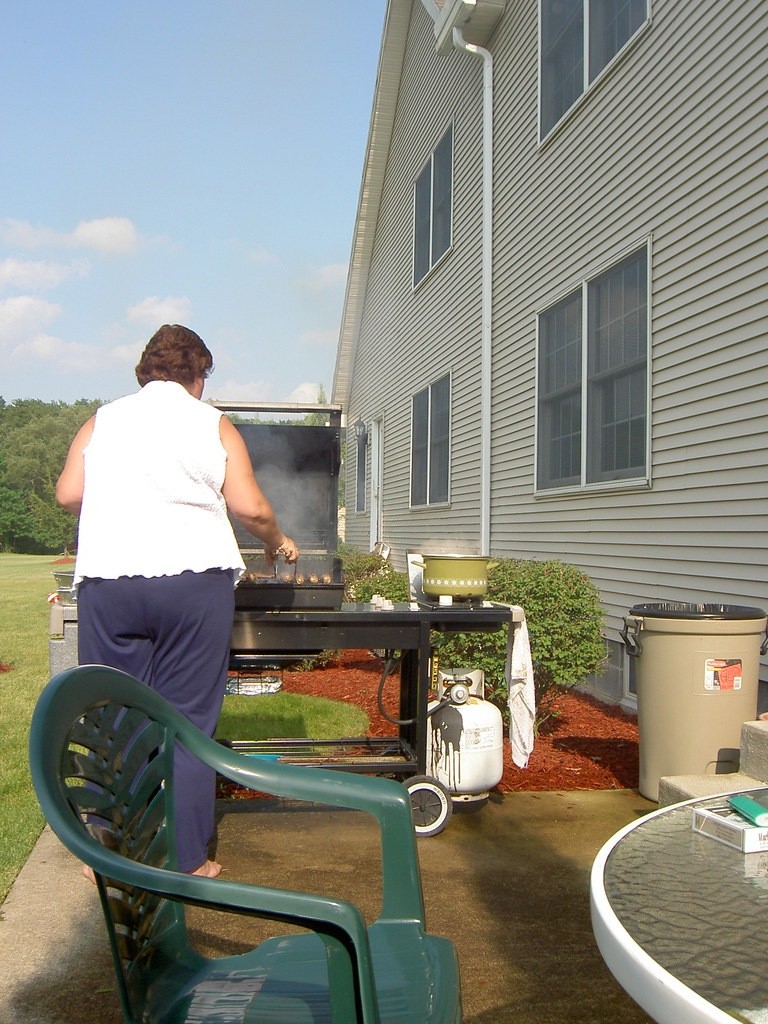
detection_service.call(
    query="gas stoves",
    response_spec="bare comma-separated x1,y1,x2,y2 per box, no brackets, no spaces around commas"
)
411,594,511,611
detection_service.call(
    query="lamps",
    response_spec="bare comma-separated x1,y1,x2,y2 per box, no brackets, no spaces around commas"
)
353,416,369,445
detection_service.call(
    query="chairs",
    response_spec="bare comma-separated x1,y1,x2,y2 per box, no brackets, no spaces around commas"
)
28,665,462,1024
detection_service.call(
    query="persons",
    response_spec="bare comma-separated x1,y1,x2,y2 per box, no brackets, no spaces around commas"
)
56,325,299,880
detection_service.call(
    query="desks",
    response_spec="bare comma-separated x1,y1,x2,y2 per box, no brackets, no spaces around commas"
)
589,786,768,1024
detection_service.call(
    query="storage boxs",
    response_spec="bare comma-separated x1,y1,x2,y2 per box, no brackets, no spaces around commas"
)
692,806,768,854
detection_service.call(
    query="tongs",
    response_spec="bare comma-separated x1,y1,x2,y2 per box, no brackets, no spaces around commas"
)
272,547,298,579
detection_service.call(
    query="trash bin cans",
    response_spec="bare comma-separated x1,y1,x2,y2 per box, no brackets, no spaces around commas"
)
619,601,768,804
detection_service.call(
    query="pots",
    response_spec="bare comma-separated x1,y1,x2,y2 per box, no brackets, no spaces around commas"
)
411,553,500,600
